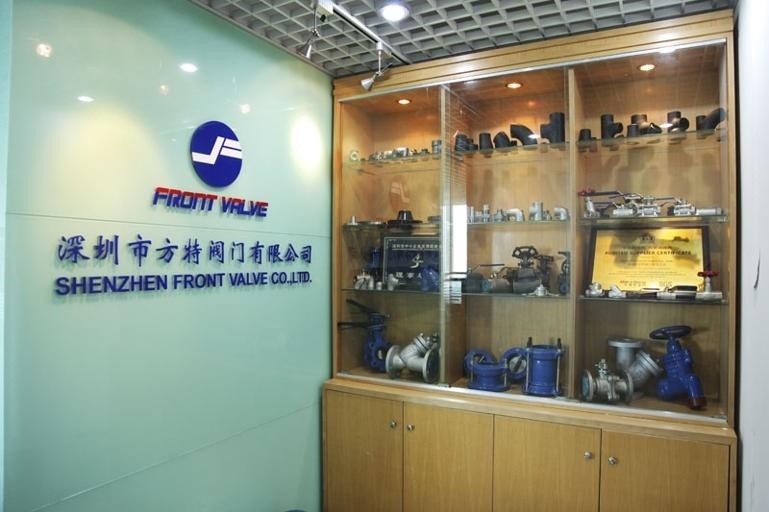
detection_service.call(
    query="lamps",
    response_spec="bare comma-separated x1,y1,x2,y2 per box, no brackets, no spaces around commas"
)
297,0,409,91
